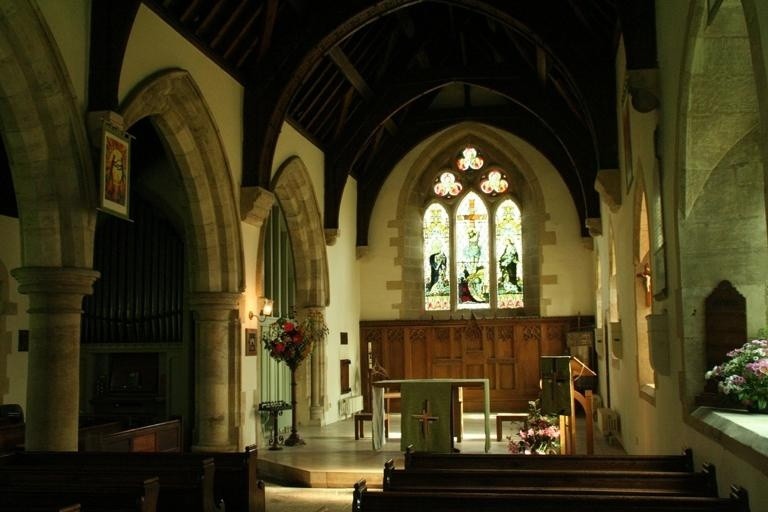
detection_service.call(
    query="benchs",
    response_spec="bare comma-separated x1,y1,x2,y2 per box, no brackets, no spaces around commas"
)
1,445,265,511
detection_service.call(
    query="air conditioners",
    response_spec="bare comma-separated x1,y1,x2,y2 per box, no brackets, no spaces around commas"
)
592,394,602,416
596,407,620,437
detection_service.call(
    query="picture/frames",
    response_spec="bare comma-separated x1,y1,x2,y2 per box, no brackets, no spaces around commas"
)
245,328,259,357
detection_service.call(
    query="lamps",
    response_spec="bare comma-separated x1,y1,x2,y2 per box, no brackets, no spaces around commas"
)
248,297,274,321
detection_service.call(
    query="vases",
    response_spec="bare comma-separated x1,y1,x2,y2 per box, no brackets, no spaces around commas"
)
748,405,766,413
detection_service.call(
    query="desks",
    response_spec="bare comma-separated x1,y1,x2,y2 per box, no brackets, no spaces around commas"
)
369,379,490,453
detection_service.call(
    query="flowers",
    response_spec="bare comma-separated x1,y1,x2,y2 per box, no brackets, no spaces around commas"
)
260,308,329,371
506,400,562,455
705,327,768,411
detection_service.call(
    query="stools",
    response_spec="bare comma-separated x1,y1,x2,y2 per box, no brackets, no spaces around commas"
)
496,413,529,442
354,412,389,441
